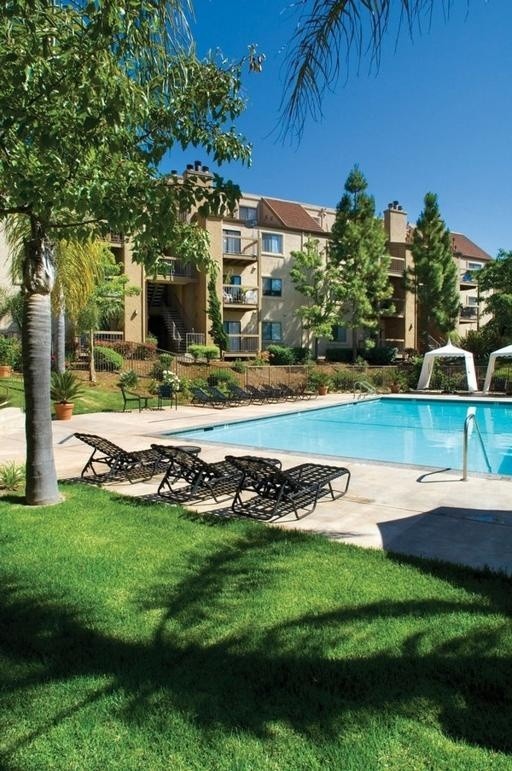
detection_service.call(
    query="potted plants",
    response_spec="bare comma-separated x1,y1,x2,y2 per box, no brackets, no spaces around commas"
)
389,372,402,394
49,371,87,421
310,369,333,396
1,334,24,378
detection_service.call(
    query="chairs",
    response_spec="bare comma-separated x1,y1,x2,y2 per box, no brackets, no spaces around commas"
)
115,382,320,412
70,432,352,526
